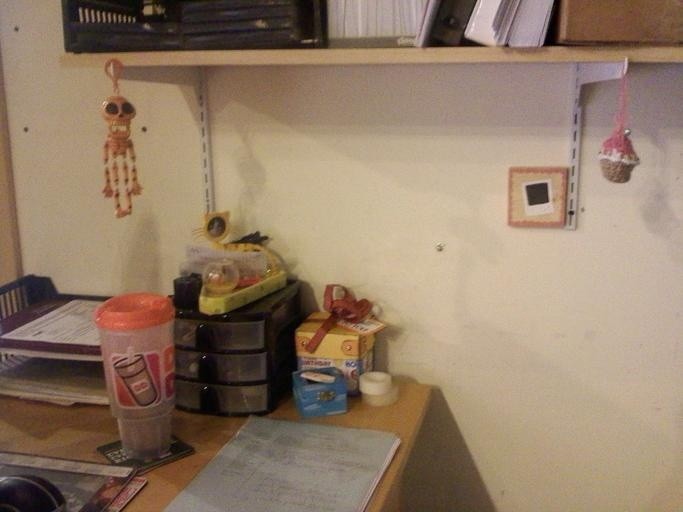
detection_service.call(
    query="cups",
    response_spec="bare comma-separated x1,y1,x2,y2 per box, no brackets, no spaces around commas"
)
94,293,178,456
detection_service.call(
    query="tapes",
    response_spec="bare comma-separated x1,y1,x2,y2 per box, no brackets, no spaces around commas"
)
361,385,399,406
358,371,392,395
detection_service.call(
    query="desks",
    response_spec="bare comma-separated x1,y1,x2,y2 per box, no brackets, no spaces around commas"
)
0,379,436,511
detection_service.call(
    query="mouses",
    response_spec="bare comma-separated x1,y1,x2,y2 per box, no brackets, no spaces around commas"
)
0,475,66,511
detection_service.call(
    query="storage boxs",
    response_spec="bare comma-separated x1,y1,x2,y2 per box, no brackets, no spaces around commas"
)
550,1,681,51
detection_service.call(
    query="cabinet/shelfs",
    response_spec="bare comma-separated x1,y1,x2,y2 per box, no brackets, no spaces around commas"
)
167,278,304,419
0,273,175,408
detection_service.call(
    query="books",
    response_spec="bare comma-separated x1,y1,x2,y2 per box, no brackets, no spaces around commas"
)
327,1,557,50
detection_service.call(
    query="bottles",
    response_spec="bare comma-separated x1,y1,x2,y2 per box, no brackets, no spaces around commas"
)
201,258,239,295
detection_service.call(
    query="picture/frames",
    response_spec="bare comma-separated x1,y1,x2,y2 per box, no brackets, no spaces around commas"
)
506,165,569,230
